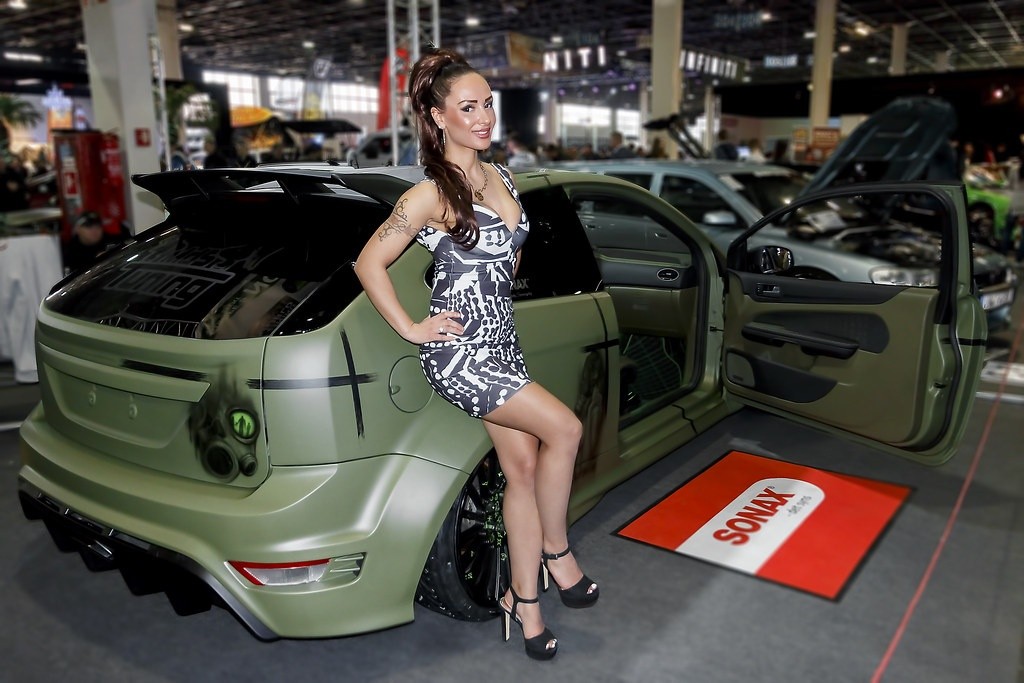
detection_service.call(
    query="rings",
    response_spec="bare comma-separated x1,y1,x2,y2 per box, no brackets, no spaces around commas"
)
439,325,443,333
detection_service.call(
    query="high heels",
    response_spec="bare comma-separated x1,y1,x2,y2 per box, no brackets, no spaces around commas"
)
540,546,599,609
498,584,559,661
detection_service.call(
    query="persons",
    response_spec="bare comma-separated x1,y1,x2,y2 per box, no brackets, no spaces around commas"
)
547,130,790,162
60,210,106,275
353,48,600,661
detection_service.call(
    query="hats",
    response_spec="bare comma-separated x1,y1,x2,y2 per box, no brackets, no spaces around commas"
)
79,211,101,226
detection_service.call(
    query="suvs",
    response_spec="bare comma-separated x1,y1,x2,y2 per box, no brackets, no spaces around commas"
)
12,96,988,642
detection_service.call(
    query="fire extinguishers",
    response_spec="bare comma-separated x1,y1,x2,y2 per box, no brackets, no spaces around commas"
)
99,125,124,187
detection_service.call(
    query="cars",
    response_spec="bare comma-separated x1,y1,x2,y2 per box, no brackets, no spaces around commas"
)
188,150,281,170
516,161,1021,336
347,128,413,167
908,161,1021,249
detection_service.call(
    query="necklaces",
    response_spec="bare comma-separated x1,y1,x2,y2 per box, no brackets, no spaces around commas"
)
471,160,488,201
